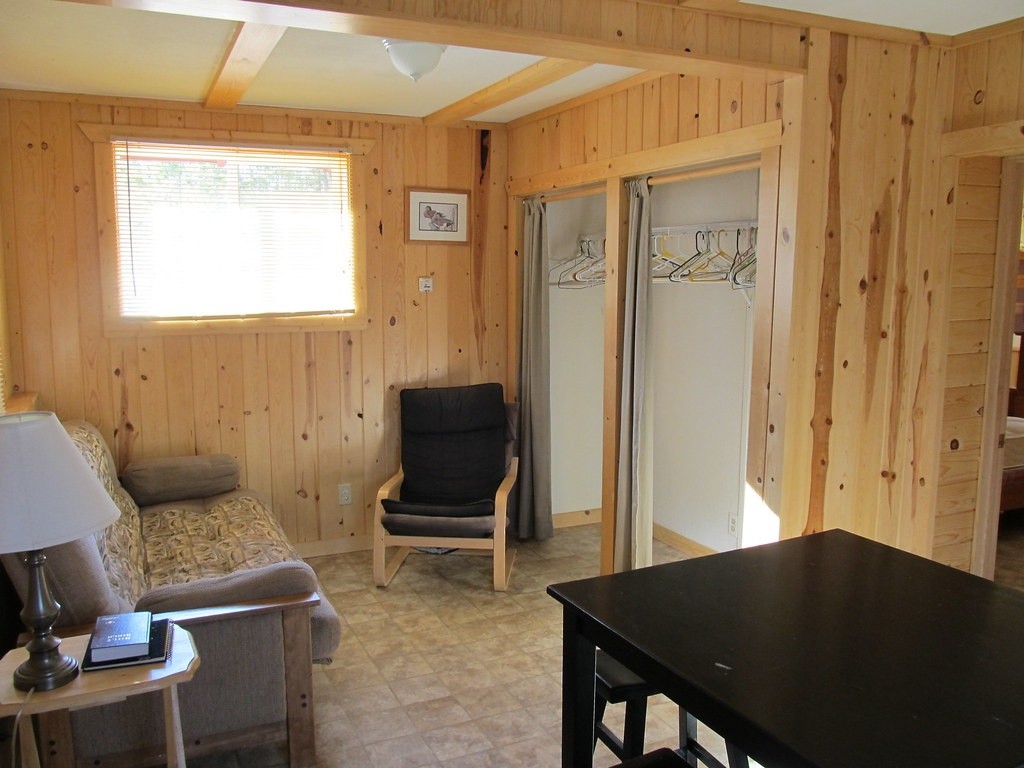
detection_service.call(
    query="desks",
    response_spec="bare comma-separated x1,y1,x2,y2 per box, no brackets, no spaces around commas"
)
546,527,1024,768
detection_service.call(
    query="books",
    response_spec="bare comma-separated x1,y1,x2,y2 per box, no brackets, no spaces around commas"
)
81,611,173,672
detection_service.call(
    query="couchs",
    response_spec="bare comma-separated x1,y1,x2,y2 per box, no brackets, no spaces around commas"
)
1,417,342,768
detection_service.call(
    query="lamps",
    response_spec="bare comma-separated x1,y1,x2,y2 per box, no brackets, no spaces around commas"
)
0,410,122,692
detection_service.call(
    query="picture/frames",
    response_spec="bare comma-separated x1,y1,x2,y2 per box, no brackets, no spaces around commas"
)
403,185,471,246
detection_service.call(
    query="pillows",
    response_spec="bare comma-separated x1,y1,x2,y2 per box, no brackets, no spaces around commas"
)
399,382,506,506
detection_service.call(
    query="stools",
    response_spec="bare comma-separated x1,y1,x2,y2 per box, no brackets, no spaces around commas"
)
591,649,750,768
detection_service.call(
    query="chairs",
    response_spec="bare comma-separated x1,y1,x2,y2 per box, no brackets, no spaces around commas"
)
374,401,520,592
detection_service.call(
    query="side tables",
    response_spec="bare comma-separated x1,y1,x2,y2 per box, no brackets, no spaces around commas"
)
0,623,202,768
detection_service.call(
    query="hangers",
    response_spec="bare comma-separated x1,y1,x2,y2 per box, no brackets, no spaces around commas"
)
547,238,607,290
649,226,759,292
